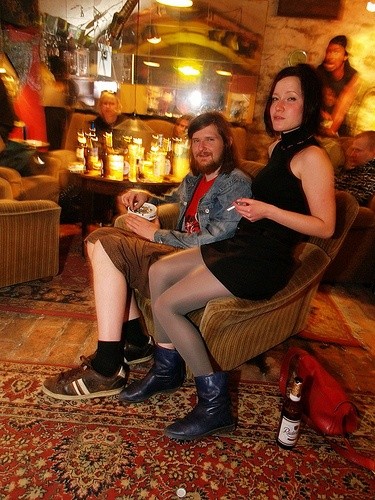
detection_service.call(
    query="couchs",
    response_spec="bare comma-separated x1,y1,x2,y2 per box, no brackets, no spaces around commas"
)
0,154,61,288
119,189,361,378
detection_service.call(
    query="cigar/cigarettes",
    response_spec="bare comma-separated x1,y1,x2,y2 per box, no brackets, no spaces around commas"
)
227,202,244,212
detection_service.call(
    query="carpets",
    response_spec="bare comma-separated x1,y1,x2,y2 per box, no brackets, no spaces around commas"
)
0,359,375,500
0,234,363,349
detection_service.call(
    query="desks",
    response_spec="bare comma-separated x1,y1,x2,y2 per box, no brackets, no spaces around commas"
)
61,164,192,251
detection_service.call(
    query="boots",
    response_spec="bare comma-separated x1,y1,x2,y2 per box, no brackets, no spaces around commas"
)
119,345,186,403
164,370,235,439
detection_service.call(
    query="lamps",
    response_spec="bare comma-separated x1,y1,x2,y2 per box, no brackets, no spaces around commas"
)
113,0,155,134
146,26,161,44
215,65,233,77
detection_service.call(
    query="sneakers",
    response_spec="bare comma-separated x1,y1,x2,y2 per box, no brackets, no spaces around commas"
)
80,335,156,368
42,354,128,399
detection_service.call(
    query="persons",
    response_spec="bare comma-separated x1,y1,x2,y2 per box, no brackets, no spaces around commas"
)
87,91,129,155
313,34,362,144
1,79,38,178
146,115,195,205
334,130,375,208
120,62,334,441
40,111,252,400
43,54,80,151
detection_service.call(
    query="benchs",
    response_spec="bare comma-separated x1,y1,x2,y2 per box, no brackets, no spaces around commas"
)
50,115,247,190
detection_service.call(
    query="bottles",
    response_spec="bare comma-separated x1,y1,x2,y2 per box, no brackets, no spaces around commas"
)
76,121,190,184
276,376,303,451
